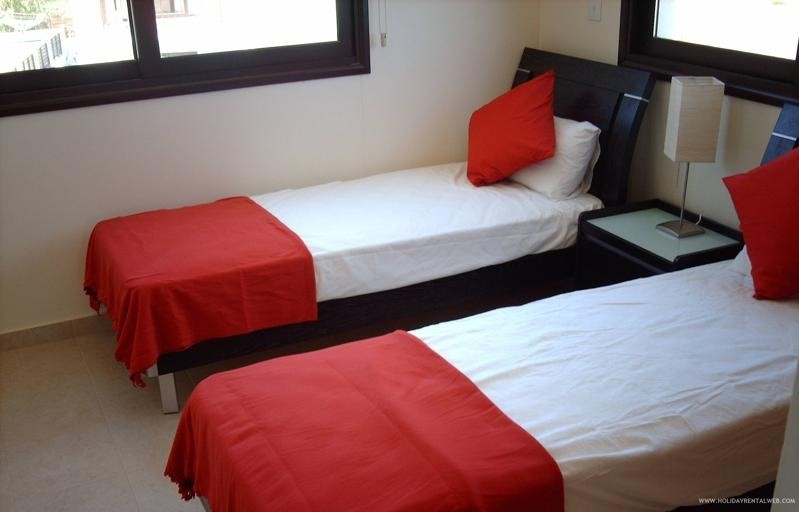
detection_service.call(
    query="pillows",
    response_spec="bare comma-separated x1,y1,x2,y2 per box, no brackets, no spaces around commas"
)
509,115,604,201
721,141,797,301
465,69,556,188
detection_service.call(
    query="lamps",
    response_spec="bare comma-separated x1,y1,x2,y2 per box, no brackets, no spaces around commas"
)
657,74,725,240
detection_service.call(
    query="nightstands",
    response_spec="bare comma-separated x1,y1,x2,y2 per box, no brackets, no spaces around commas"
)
574,198,745,288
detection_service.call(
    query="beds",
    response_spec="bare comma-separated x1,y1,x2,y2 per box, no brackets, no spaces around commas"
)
82,45,657,417
161,103,799,512
